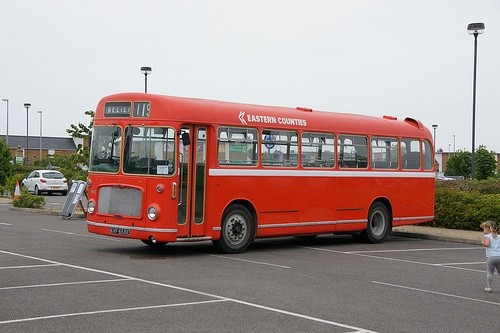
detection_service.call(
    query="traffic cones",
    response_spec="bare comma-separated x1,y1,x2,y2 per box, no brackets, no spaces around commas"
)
9,180,20,204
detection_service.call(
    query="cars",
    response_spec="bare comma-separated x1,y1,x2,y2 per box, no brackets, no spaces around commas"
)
21,170,69,196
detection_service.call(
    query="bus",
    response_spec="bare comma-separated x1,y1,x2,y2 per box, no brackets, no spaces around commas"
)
84,91,436,254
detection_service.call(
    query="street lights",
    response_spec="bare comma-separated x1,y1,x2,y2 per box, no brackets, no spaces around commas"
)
24,103,32,164
37,110,43,161
432,124,438,150
140,66,152,93
468,23,486,181
2,99,9,148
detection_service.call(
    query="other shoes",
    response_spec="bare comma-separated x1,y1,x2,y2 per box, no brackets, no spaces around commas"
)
483,287,492,293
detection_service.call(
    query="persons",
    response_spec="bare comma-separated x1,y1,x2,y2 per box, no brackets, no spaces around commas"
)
480,221,500,292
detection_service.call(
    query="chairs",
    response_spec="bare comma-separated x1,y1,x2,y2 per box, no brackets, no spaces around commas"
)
304,158,407,168
